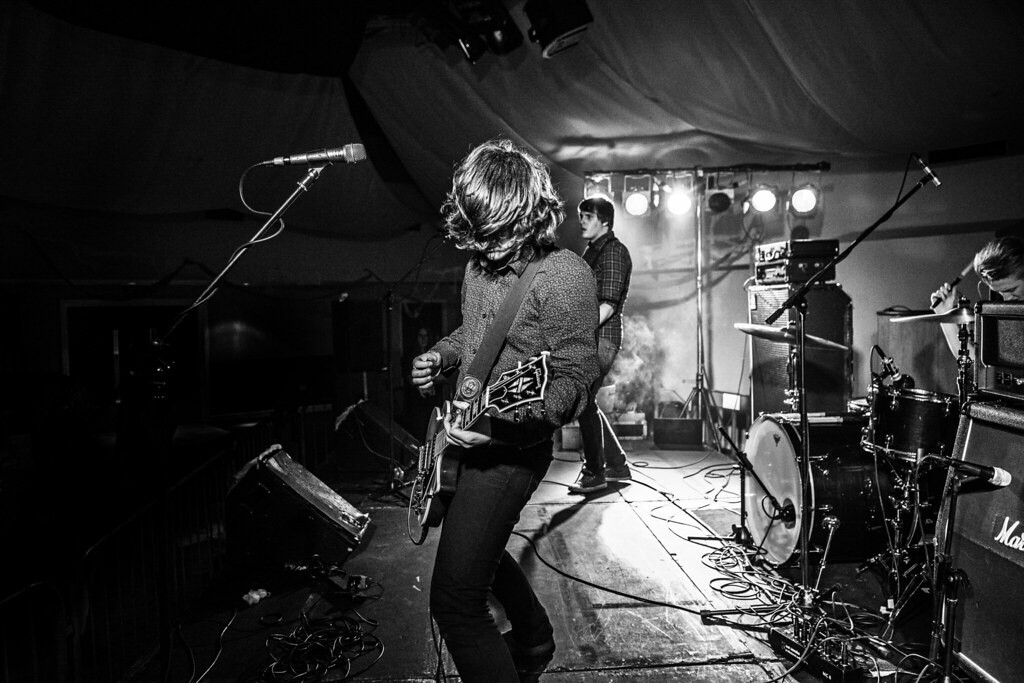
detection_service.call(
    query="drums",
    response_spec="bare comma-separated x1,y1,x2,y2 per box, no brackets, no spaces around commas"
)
860,386,960,465
739,411,872,566
846,396,871,413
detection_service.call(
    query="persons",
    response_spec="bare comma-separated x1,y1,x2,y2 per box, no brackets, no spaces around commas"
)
400,326,435,442
930,236,1024,361
412,142,600,683
569,198,632,493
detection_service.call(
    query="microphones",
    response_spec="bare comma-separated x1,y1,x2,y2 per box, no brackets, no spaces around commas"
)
876,345,902,381
912,153,942,187
942,456,1011,485
780,505,796,521
263,143,366,167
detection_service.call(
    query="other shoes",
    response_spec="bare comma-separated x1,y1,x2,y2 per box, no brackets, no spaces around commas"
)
501,627,556,658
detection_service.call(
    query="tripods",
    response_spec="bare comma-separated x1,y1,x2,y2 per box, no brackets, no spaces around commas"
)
812,454,937,636
701,175,930,658
687,426,783,576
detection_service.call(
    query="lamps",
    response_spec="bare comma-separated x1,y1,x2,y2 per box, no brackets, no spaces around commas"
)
584,163,827,216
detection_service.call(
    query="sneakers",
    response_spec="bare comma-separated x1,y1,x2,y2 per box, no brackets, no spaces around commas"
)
604,464,632,481
567,474,608,491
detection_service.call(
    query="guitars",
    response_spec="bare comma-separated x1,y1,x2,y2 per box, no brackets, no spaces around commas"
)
416,349,555,527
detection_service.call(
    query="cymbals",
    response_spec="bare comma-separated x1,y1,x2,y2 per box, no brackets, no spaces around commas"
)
889,307,976,324
732,321,851,351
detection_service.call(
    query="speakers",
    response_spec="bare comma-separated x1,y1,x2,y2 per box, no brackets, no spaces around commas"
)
747,280,854,424
334,399,422,472
929,397,1024,683
223,443,372,583
